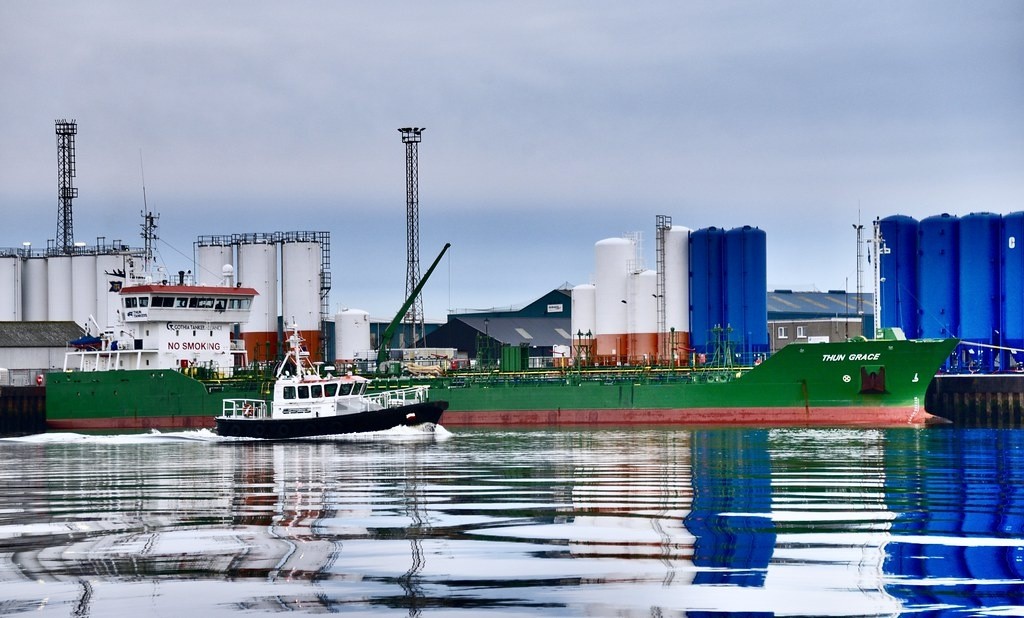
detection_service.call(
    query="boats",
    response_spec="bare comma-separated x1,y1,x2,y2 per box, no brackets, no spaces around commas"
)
210,315,450,446
70,335,110,351
41,214,963,433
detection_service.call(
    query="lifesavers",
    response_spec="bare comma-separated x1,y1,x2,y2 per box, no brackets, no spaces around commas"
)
243,404,253,417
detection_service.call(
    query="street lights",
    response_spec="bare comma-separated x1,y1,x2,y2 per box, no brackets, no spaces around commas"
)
484,317,490,359
851,223,863,318
